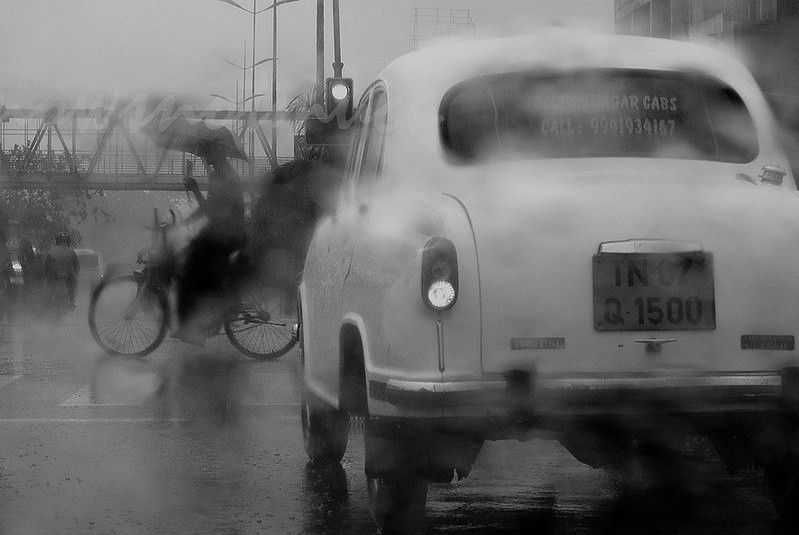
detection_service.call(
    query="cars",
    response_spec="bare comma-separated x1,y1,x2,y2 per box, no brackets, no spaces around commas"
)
297,34,799,531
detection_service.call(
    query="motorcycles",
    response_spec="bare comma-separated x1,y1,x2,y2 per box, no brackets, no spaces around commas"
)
48,272,76,326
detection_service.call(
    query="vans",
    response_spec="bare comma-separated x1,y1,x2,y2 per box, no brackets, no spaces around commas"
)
76,249,106,287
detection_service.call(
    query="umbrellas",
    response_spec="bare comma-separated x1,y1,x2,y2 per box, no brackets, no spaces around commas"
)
139,114,248,178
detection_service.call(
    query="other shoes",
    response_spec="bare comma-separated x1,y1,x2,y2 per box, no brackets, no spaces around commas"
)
171,327,206,349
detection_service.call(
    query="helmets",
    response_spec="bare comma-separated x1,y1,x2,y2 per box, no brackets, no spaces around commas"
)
56,233,71,247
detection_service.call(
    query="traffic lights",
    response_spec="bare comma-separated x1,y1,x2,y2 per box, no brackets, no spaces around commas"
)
325,78,353,123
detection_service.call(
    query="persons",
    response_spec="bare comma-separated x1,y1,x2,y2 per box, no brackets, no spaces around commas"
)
0,222,80,311
170,138,247,339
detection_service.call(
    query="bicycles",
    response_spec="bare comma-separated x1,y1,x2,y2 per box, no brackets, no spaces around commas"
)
89,209,300,358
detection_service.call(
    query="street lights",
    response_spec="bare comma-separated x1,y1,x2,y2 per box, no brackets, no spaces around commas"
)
206,1,297,197
